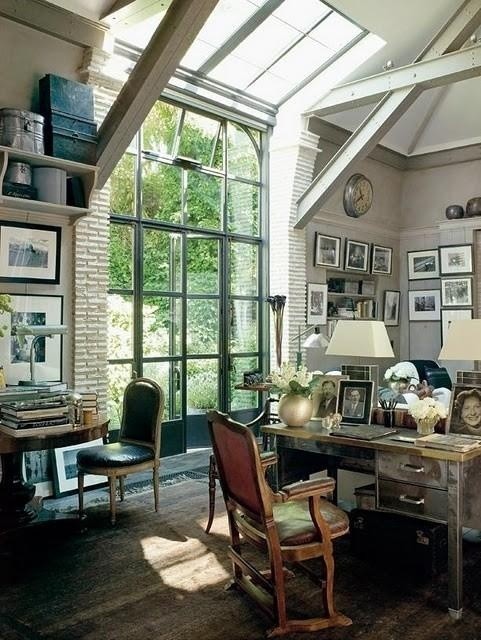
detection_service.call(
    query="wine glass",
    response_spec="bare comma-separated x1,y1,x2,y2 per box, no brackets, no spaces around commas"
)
332,415,342,429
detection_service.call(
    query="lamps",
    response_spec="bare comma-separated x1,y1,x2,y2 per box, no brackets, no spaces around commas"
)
15,326,68,386
291,322,330,371
325,320,395,407
438,318,481,386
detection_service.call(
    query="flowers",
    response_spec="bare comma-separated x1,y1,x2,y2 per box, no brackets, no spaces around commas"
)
267,366,323,398
407,396,448,422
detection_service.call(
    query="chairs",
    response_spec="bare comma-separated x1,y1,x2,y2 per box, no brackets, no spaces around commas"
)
203,407,354,640
76,377,165,526
205,397,296,534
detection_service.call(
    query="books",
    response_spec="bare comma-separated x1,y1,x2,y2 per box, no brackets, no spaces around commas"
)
329,425,399,440
415,433,481,453
0,380,99,439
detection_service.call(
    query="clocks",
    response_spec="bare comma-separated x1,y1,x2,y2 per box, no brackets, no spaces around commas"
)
343,173,374,218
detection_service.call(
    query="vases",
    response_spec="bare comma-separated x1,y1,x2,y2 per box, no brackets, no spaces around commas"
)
277,394,313,427
413,417,437,435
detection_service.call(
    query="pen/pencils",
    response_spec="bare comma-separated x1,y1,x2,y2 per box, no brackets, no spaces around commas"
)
378,398,398,409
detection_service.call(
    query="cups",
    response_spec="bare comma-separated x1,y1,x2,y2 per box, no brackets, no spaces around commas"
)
322,418,332,429
84,410,92,425
384,409,395,427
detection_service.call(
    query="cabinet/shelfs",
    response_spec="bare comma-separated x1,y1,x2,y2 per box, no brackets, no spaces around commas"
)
260,415,481,624
0,145,101,227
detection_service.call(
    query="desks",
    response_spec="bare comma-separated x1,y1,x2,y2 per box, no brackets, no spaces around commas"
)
0,412,111,481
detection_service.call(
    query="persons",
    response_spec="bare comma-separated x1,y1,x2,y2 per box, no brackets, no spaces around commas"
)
344,387,365,418
453,388,481,436
316,381,336,418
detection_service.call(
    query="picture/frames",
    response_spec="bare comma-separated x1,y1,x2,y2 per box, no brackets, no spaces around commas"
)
0,220,62,285
50,436,110,498
335,378,375,427
445,383,481,441
0,292,64,383
309,373,349,421
305,230,474,349
